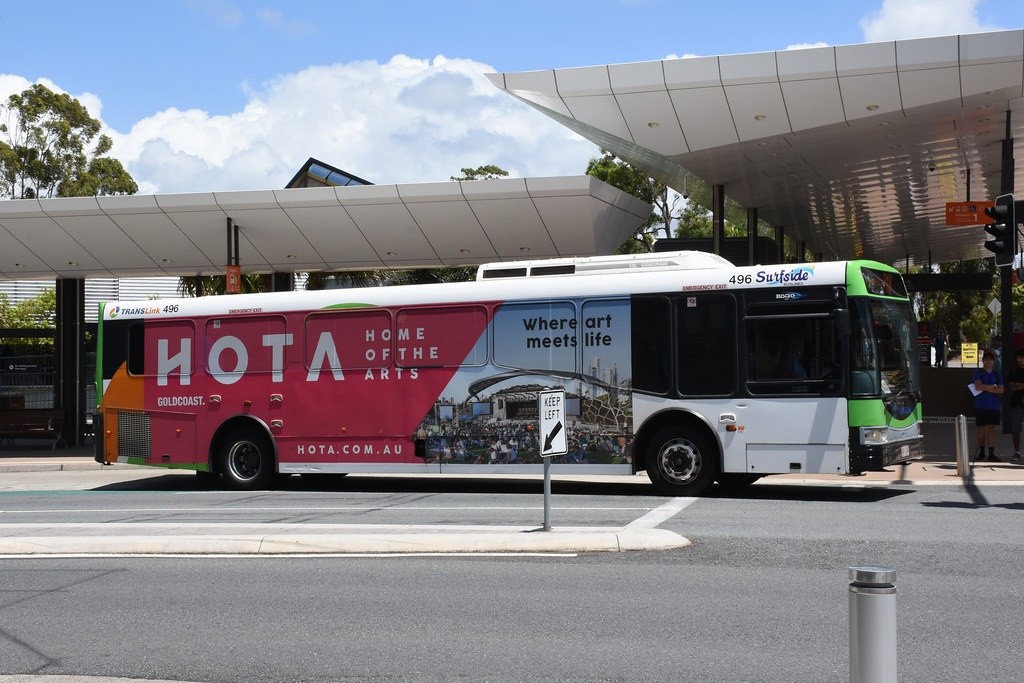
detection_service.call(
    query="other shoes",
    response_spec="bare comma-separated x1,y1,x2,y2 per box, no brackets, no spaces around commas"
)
1011,453,1020,461
934,363,937,366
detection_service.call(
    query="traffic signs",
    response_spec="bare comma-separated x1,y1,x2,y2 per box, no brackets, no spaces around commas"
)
539,389,569,456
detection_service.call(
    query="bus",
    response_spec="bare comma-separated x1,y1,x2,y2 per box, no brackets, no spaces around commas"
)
95,249,946,497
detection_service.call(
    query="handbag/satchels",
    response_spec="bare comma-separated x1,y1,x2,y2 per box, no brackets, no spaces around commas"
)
931,337,937,346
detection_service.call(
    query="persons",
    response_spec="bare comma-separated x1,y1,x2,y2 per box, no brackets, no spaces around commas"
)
971,352,1005,462
417,418,631,464
1004,351,1024,461
933,321,948,369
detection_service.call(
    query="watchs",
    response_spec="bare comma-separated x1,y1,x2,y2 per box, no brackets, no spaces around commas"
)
994,383,998,388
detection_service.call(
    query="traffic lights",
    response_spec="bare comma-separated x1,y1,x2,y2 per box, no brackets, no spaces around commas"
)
983,194,1016,267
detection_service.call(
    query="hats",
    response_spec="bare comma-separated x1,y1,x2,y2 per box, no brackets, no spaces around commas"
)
991,335,1002,342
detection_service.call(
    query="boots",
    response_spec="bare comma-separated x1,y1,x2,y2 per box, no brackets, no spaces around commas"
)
987,448,1001,461
973,446,986,461
